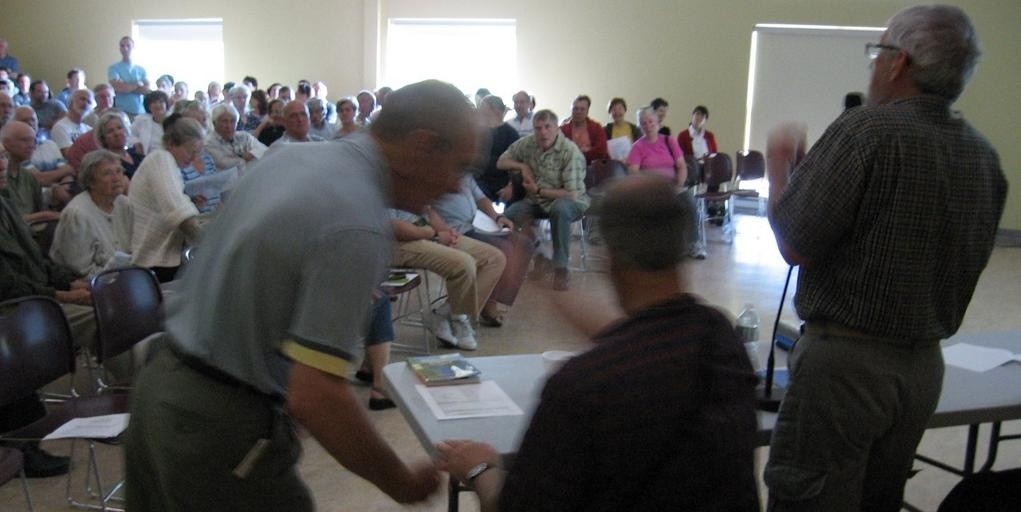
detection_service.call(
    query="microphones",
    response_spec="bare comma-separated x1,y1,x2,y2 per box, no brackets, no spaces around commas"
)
842,92,863,112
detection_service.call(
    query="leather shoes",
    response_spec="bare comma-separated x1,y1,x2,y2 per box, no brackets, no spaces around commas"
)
481,300,508,325
708,206,725,226
356,361,373,382
22,448,71,478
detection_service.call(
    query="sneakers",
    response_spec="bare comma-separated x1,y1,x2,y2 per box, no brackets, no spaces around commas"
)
553,267,571,290
688,241,706,259
422,308,477,350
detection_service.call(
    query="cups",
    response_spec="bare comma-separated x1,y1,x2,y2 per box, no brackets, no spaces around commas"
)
541,350,575,377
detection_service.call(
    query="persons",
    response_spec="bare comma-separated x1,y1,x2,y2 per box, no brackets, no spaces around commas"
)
1,141,93,479
763,4,1009,512
124,80,483,511
842,90,863,109
432,172,764,511
1,32,727,411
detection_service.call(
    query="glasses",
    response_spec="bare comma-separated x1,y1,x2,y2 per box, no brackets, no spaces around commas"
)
863,41,901,59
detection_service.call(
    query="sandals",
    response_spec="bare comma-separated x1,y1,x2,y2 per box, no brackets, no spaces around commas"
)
369,387,396,410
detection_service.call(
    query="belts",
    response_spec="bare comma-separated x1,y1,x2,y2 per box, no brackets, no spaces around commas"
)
167,342,242,386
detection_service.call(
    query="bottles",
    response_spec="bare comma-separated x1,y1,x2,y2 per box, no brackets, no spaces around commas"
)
733,301,761,373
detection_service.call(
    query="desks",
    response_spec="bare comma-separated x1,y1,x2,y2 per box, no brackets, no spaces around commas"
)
380,330,1021,512
